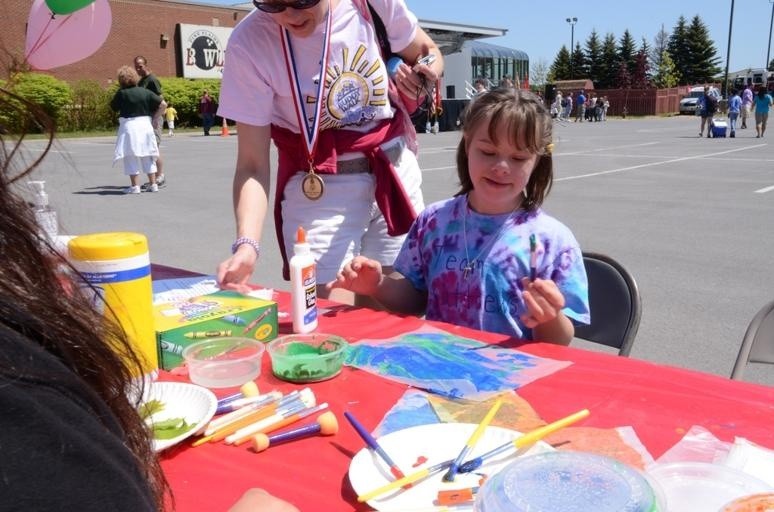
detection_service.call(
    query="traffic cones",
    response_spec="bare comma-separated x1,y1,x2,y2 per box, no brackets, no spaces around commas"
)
219,118,231,137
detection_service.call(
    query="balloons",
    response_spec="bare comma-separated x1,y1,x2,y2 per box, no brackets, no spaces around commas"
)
24,0,113,70
44,0,93,18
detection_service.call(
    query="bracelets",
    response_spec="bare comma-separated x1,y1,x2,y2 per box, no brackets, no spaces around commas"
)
231,238,261,256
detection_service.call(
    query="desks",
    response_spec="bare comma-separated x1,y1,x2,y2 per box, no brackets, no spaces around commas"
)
58,261,774,512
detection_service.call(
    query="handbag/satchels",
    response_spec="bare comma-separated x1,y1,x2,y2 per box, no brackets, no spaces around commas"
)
696,107,701,116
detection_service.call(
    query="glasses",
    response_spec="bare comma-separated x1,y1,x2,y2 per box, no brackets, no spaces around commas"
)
253,0,320,13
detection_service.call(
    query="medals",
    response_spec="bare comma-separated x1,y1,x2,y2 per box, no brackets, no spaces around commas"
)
302,173,324,200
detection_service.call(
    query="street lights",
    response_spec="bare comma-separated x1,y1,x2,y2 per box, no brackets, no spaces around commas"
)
566,17,577,79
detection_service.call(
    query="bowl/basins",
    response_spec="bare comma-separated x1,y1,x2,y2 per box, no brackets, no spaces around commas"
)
181,336,266,389
265,332,349,385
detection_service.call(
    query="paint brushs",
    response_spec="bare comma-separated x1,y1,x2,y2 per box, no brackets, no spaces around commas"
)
527,235,538,340
192,388,327,446
344,399,591,504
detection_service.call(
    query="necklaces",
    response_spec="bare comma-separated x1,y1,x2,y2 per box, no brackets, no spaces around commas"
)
460,194,524,282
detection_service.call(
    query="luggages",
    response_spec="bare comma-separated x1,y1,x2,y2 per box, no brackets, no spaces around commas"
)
711,117,727,138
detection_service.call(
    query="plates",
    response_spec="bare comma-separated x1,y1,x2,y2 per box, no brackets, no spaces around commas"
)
350,422,556,510
130,377,216,456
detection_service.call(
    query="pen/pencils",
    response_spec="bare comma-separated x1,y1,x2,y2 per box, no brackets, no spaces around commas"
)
208,341,245,360
185,331,232,339
160,340,185,356
225,315,243,325
243,308,273,333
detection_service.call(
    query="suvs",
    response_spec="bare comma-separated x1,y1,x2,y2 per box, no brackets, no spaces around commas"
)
678,86,722,116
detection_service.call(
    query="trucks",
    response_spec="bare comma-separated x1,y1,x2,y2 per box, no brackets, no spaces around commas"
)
734,69,768,93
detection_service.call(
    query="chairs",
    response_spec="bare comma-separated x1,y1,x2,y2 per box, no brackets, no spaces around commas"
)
573,251,643,356
730,302,774,385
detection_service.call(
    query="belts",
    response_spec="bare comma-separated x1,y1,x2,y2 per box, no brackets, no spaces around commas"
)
336,146,402,173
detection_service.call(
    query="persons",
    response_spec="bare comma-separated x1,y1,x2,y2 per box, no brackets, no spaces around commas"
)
134,55,165,188
695,82,774,138
476,72,611,121
110,65,167,194
217,4,445,309
199,90,214,136
164,103,179,136
0,46,175,511
325,87,592,347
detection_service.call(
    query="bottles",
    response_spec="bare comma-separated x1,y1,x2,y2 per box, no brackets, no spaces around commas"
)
67,231,159,393
285,227,319,335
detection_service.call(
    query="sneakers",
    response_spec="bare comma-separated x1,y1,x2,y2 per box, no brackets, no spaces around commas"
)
123,185,140,193
141,175,165,192
